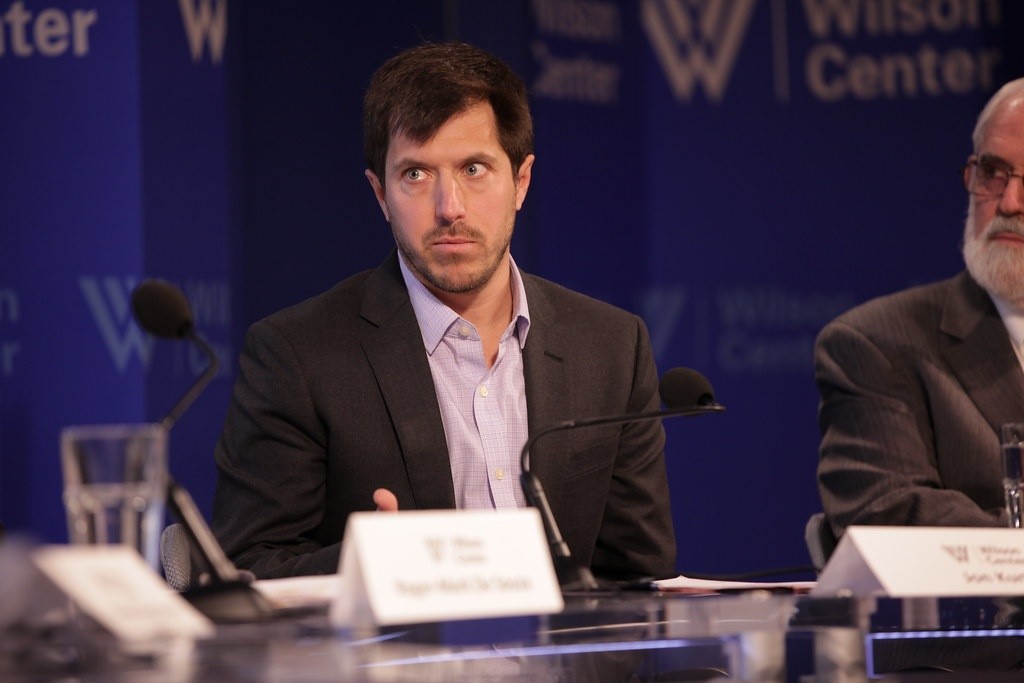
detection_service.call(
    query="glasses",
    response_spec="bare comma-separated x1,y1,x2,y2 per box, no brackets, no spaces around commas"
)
959,154,1024,196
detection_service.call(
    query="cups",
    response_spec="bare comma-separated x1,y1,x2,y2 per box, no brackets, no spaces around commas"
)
1001,420,1024,531
60,426,170,582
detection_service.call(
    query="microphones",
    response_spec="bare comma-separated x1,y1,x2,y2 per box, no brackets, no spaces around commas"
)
127,273,287,624
518,368,726,598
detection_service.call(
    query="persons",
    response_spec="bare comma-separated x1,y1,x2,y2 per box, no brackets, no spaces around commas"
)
812,77,1024,559
207,41,679,588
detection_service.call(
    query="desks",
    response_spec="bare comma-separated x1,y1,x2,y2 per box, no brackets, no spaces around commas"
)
151,583,1024,683
